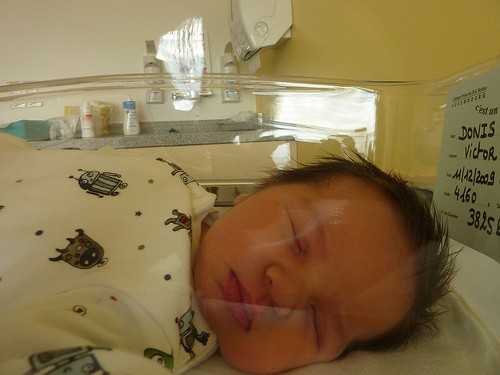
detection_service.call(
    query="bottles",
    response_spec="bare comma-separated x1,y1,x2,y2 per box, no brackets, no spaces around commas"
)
123,91,140,135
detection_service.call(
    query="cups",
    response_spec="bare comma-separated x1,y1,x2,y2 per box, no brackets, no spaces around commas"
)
92,107,113,134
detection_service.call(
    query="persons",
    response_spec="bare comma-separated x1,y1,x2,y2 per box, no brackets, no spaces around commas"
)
0,129,465,375
